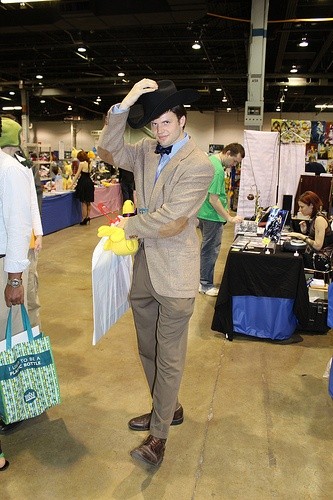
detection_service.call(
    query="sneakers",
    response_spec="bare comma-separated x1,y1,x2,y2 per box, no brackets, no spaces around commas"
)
198,283,219,296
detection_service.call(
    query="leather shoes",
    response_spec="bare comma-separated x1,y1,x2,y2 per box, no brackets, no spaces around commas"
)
128,404,184,431
130,435,167,467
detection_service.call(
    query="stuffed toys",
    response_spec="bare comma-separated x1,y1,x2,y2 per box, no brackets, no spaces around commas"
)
62,163,74,190
98,200,139,257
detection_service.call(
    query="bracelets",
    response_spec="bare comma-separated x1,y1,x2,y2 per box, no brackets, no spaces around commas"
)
304,236,310,242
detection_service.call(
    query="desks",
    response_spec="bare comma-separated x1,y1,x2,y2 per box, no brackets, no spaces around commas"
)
89,184,122,219
209,233,312,345
295,174,333,218
40,190,83,236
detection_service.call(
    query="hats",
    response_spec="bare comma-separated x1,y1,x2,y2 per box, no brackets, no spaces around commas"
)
127,80,202,129
0,117,34,169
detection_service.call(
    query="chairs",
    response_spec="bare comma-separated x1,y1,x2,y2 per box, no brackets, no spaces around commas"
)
302,267,333,306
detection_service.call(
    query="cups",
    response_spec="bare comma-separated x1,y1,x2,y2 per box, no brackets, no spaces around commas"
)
240,221,257,233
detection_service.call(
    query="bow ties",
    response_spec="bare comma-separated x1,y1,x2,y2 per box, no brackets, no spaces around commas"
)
154,144,173,155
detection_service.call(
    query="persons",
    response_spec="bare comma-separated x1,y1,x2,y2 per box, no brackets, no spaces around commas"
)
286,191,333,280
305,155,326,175
118,167,134,205
96,78,215,467
69,150,94,225
197,143,244,296
0,114,42,431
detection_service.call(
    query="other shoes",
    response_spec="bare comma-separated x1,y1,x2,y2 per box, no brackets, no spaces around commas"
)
0,419,25,433
0,459,10,471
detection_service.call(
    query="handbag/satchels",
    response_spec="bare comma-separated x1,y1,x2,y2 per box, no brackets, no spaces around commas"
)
92,214,134,345
262,207,289,245
0,303,62,425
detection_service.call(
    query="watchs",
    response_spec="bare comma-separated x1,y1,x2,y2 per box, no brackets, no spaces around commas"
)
7,279,22,288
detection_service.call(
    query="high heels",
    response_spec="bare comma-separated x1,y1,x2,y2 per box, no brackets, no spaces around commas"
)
80,217,90,225
